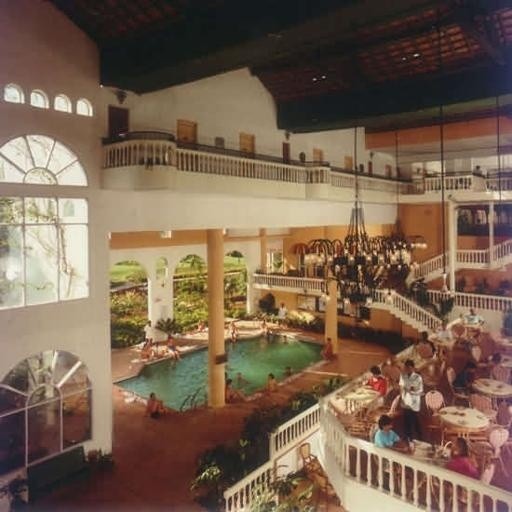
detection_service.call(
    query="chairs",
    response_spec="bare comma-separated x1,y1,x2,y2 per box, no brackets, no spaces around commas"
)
421,362,447,390
425,391,447,422
471,429,511,480
445,464,496,512
446,369,479,412
368,425,398,481
369,394,400,421
469,394,501,425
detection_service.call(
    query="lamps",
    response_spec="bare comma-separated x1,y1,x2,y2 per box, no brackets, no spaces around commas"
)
290,49,428,303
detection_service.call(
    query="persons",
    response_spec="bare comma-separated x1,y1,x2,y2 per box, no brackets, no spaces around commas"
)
278,303,286,317
389,355,405,371
454,361,478,395
444,436,480,485
236,371,243,384
321,338,334,360
228,321,239,343
283,367,293,376
438,324,451,338
473,166,483,174
196,318,205,331
282,336,288,342
225,379,248,403
417,331,434,357
366,365,388,408
398,361,427,439
266,374,277,391
372,416,409,457
260,319,269,339
460,308,485,338
143,393,169,419
476,332,497,361
486,353,503,381
134,321,181,363
